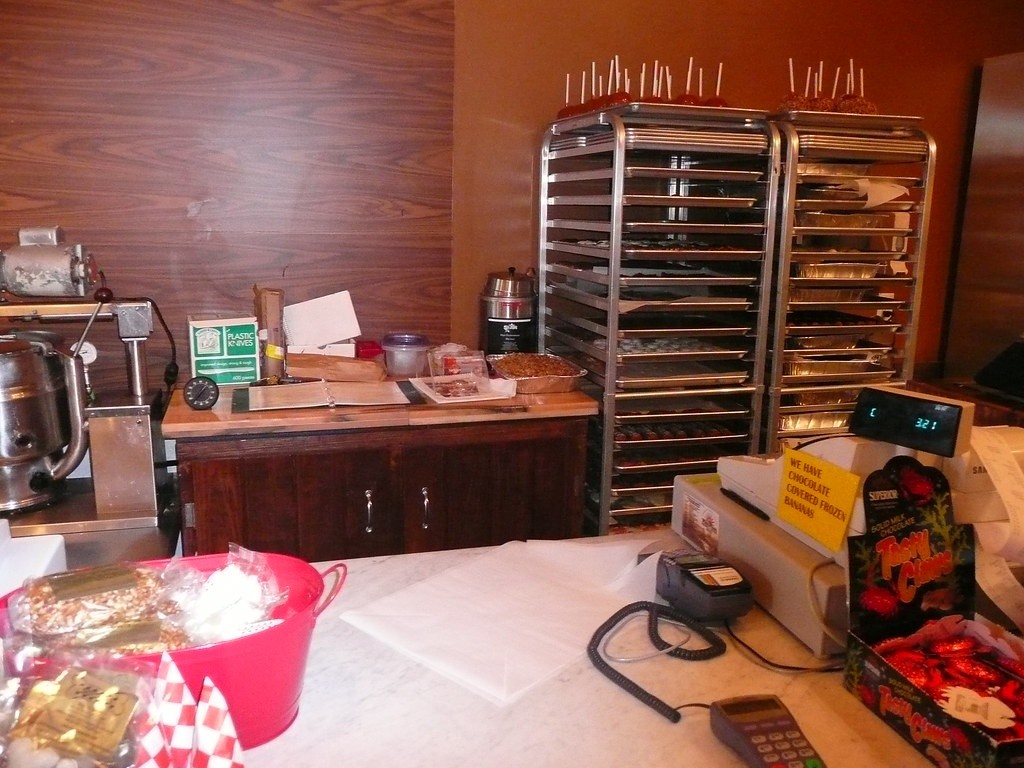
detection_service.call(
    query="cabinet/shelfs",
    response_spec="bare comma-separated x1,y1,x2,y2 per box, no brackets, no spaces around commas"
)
175,414,586,563
537,114,938,536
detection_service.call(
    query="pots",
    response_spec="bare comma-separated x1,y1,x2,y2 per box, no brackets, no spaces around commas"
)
485,267,534,297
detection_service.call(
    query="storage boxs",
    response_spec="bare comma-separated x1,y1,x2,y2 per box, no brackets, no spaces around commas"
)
843,454,1023,767
485,352,589,395
188,316,260,385
253,283,285,382
381,332,431,378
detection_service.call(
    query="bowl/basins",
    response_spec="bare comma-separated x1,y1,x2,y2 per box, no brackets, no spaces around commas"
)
380,340,430,376
482,294,536,319
0,552,347,751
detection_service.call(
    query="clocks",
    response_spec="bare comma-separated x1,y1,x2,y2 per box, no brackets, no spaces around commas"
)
184,376,219,409
